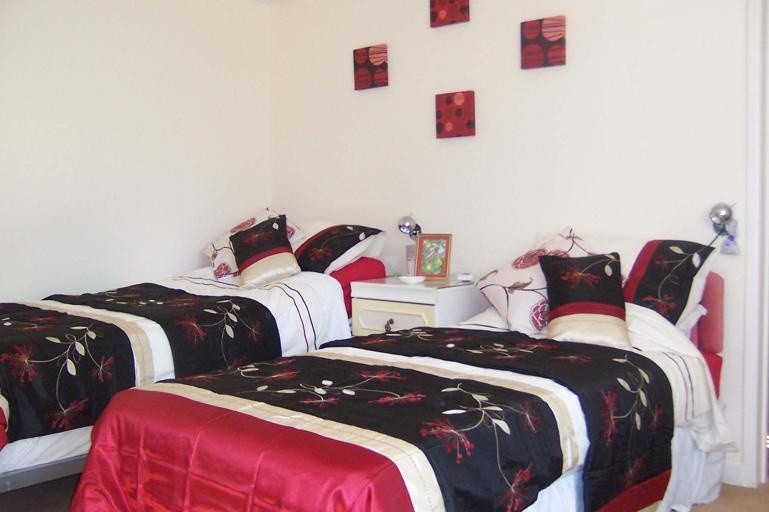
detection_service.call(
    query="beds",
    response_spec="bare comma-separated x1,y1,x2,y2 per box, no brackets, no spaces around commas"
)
68,271,741,512
0,256,386,493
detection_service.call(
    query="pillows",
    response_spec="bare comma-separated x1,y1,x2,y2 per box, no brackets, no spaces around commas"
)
474,225,716,349
202,207,385,290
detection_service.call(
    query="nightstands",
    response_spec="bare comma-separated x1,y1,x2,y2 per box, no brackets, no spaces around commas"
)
350,275,492,337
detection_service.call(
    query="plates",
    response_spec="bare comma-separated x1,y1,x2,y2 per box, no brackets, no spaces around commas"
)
398,276,425,284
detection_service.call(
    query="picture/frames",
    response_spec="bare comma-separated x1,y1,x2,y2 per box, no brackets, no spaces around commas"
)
414,233,452,280
